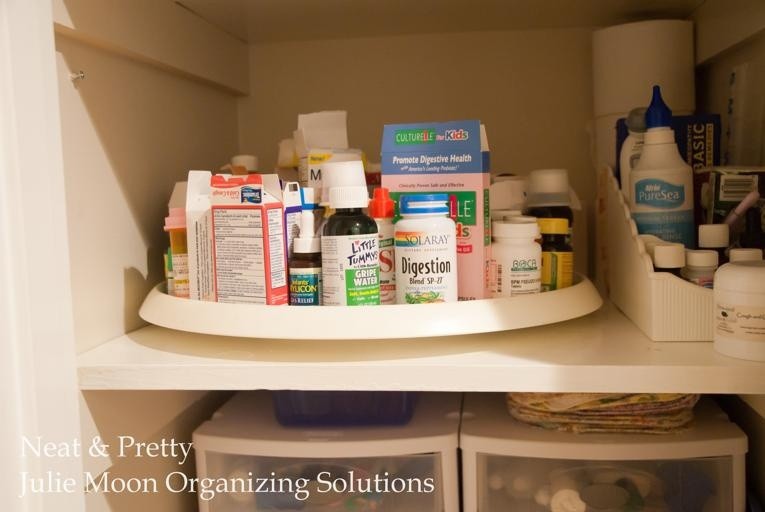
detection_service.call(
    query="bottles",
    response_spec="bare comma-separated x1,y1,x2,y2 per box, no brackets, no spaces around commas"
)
320,183,382,306
710,245,765,363
526,167,575,247
491,208,575,298
609,85,732,290
290,235,323,306
392,192,457,305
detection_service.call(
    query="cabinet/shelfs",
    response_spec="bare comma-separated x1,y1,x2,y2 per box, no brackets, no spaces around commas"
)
0,2,765,512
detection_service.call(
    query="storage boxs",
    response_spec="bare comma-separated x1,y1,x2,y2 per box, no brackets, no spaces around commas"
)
189,391,460,511
459,391,749,512
